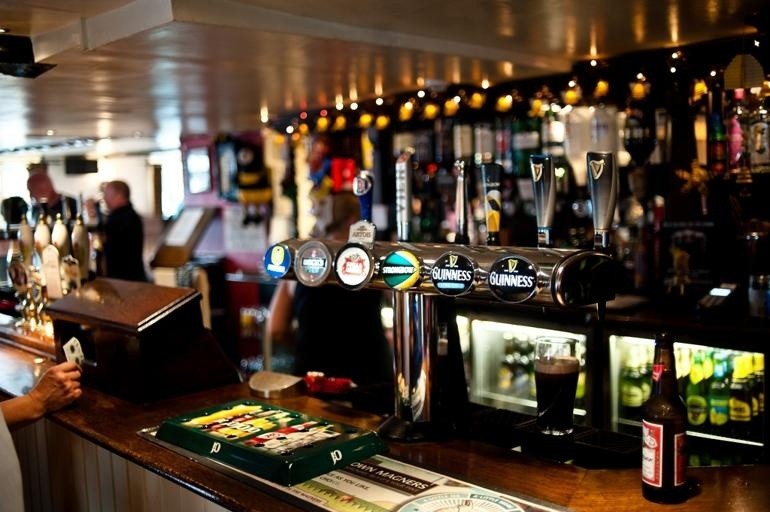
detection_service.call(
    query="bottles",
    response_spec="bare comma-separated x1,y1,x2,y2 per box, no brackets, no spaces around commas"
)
497,330,586,407
641,330,690,503
389,79,769,286
619,341,764,437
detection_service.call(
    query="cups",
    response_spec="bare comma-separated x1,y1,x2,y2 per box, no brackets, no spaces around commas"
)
534,337,581,435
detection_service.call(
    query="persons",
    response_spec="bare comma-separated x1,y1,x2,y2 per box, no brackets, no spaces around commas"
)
263,192,395,415
101,180,148,281
26,172,77,226
0,361,85,432
